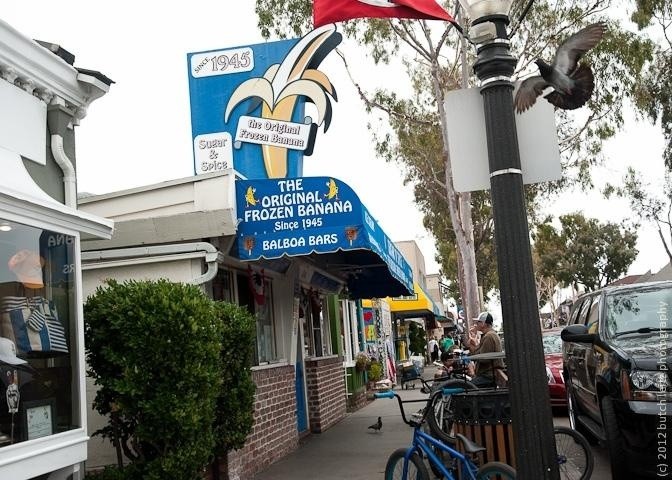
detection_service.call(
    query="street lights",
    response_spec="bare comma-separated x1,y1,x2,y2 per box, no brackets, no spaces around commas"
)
458,0,557,477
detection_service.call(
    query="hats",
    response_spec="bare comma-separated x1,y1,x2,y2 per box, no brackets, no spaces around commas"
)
472,311,493,324
0,337,28,365
8,248,45,289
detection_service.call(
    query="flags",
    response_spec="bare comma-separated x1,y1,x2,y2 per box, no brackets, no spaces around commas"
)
310,0,466,36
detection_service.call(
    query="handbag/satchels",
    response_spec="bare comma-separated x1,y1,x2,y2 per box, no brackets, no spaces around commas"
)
0,296,70,358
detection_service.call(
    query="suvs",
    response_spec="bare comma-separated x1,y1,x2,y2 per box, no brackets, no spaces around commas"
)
540,281,671,480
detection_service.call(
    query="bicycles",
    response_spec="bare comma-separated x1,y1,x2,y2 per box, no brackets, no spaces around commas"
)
374,367,595,479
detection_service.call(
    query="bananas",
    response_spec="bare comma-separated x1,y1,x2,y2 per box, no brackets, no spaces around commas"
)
247,187,255,205
330,180,335,197
225,22,338,178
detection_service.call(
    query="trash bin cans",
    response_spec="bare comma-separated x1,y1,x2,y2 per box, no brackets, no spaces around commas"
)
411,357,424,374
453,390,516,480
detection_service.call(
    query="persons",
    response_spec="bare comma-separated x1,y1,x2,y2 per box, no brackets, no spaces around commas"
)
466,312,504,379
412,330,475,419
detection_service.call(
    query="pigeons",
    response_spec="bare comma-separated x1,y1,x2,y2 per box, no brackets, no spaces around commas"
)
513,23,608,115
368,417,382,432
